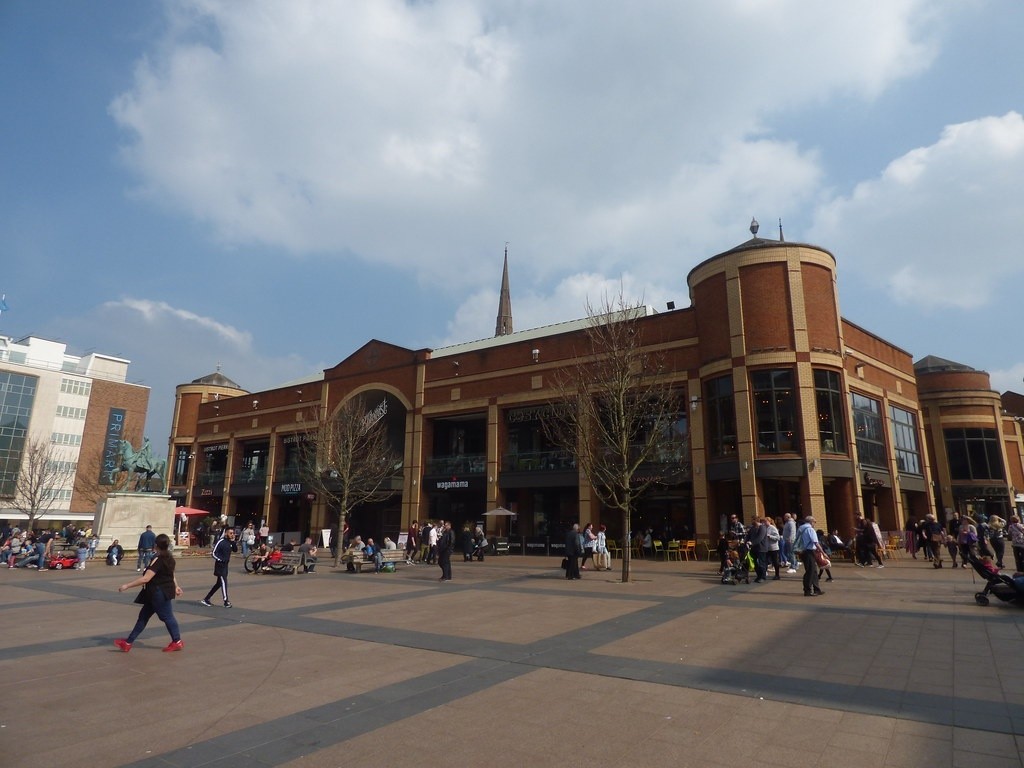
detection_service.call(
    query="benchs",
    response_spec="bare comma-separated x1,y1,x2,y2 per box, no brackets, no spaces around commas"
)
54,539,73,550
339,548,412,573
260,551,318,576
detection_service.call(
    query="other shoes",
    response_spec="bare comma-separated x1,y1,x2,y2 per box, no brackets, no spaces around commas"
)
441,577,451,582
136,568,141,572
409,559,415,566
37,567,49,571
7,565,18,569
854,554,1005,570
751,562,836,596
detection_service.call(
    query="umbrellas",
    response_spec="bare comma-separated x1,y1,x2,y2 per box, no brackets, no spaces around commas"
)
175,505,210,532
481,505,516,536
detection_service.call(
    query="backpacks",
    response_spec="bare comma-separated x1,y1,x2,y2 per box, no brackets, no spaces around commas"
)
792,528,813,555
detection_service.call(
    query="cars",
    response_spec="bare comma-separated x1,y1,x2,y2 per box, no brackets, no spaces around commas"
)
47,555,79,570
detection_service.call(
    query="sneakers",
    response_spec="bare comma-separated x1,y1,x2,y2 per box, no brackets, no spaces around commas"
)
113,638,133,653
201,598,213,606
162,638,184,653
223,602,232,608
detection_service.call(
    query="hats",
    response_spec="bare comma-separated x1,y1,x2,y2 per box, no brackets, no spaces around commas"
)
963,515,977,525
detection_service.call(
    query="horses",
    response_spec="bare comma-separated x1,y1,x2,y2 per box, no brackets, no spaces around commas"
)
109,440,166,494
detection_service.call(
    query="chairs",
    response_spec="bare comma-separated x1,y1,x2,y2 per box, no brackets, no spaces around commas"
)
878,536,905,563
468,461,486,473
605,540,718,561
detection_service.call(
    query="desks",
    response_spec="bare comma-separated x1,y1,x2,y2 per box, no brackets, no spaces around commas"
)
521,459,532,470
558,458,568,469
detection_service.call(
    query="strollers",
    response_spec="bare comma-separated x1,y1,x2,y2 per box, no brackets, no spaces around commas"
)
958,551,1024,606
721,543,752,585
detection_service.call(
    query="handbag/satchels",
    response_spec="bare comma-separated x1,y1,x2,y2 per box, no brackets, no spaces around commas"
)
591,538,598,553
479,538,488,547
560,554,570,569
381,561,396,573
133,589,152,604
246,532,256,546
593,551,608,569
821,544,832,559
746,551,758,572
813,552,832,569
930,533,943,543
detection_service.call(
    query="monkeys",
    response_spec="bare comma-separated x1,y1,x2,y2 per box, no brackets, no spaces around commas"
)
384,537,396,550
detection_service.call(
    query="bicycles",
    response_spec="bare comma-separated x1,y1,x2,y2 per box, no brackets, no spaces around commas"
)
244,553,286,572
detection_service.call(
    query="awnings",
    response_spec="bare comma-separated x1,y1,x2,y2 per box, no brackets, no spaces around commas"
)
0,511,95,520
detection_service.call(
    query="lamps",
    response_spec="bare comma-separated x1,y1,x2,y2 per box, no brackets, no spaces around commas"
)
856,462,861,470
695,466,699,474
1010,485,1014,492
213,393,220,401
896,475,901,481
744,462,748,470
489,476,493,482
930,481,934,486
944,486,948,491
186,488,190,494
223,487,227,492
188,455,194,459
667,301,675,311
855,362,865,373
813,459,817,467
265,485,269,490
691,395,698,410
413,480,417,485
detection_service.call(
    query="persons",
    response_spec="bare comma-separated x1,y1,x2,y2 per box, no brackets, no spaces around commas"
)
905,512,1024,579
138,525,155,570
238,520,269,557
194,519,230,548
279,538,296,567
0,523,99,571
565,523,611,580
298,537,318,573
114,534,184,652
717,512,848,596
851,511,884,569
133,435,153,468
384,537,396,550
635,528,652,556
406,520,455,581
463,524,489,562
249,543,281,575
201,527,239,607
107,539,124,565
329,516,385,574
